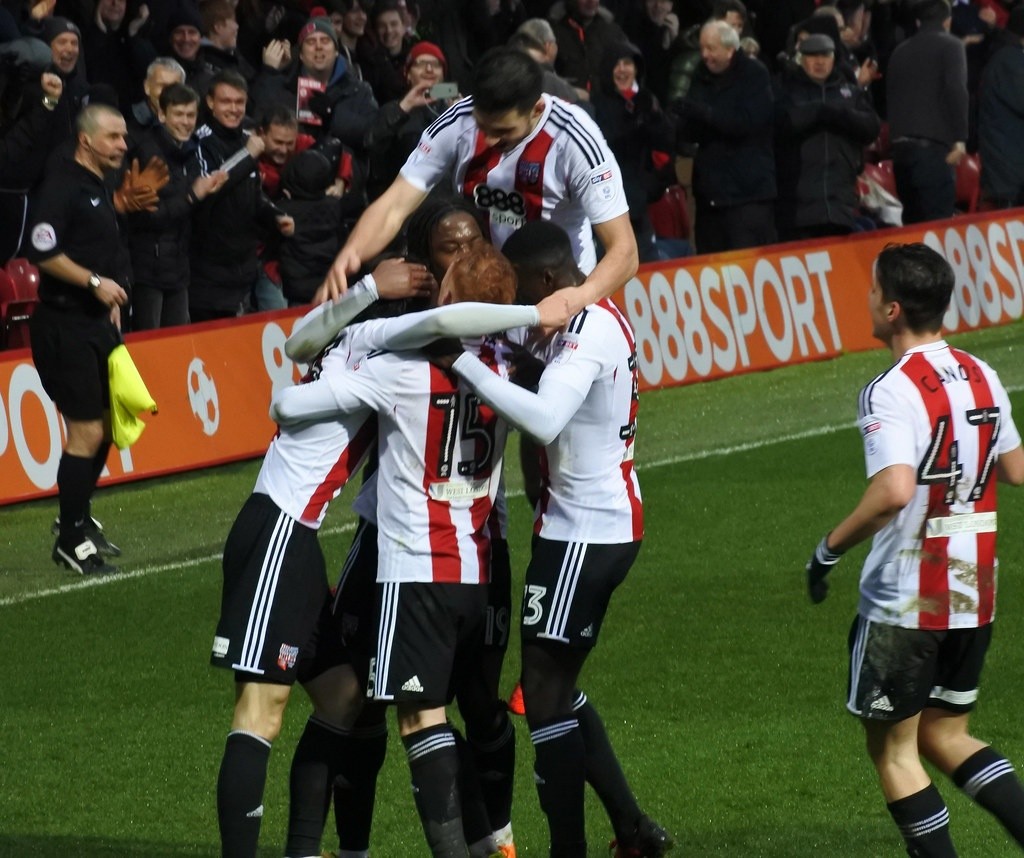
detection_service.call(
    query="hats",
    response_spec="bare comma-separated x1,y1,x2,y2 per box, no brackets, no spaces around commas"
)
298,6,338,53
168,6,204,37
41,16,81,48
402,40,448,81
800,33,836,54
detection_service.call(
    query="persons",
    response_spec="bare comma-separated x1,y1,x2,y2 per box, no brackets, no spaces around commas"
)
26,103,129,576
0,0,1024,858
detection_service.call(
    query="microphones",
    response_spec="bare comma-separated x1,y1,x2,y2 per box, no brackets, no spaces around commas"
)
86,143,115,162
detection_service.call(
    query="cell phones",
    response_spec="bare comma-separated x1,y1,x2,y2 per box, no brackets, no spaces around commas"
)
430,83,458,98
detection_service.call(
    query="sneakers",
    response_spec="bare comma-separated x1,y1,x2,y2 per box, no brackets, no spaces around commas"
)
52,533,105,578
610,816,673,858
51,513,123,561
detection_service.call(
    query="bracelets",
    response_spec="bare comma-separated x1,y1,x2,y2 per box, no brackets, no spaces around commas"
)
191,187,200,204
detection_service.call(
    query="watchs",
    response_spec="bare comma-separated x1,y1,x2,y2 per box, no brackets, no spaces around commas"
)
89,273,101,292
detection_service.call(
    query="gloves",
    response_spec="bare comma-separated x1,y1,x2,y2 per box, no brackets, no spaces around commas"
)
819,101,841,129
806,532,844,604
672,97,711,125
